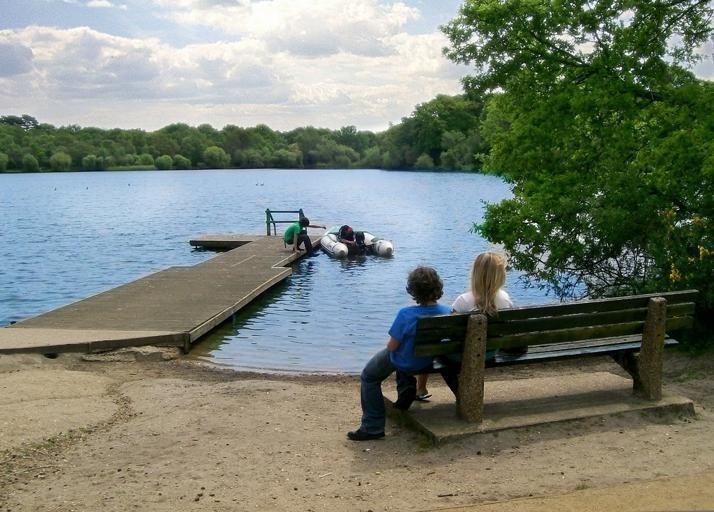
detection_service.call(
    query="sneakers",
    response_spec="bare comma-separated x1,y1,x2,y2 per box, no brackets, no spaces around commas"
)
392,385,417,410
347,428,386,441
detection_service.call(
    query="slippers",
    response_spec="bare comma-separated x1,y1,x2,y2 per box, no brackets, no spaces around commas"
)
414,393,433,400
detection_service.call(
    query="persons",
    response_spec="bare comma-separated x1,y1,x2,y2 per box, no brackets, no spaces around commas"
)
283,217,320,257
440,251,515,406
339,225,354,241
347,265,453,441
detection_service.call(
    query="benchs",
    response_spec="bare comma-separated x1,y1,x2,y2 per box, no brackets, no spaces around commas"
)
414,289,699,423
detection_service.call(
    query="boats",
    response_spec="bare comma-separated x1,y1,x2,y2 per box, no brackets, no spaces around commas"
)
321,224,393,258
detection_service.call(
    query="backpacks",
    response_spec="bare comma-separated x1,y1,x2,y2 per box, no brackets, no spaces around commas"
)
337,224,354,242
